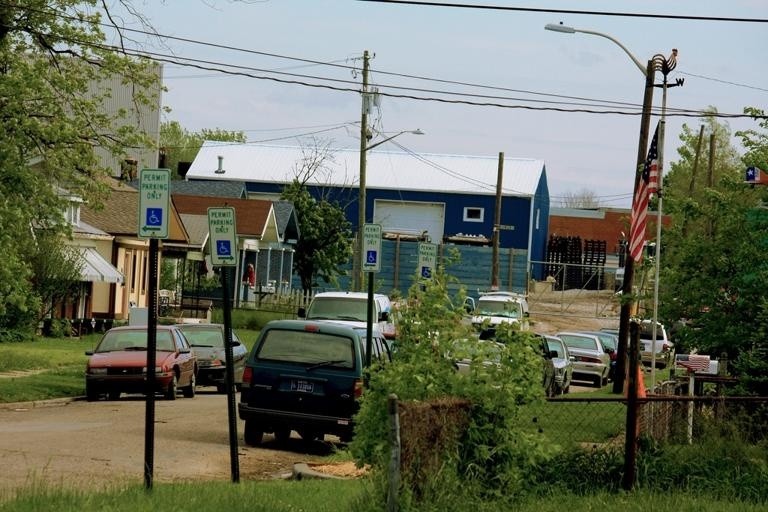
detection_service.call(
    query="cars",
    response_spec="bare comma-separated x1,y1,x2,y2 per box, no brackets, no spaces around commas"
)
177,322,248,392
436,317,677,398
83,324,198,402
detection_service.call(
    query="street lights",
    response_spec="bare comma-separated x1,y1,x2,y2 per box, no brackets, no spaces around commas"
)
540,20,657,396
352,125,426,292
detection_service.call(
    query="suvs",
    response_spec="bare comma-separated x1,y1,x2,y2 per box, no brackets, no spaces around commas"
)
463,288,530,337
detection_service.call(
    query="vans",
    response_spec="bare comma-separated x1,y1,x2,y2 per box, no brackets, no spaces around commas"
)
239,317,393,448
297,291,395,333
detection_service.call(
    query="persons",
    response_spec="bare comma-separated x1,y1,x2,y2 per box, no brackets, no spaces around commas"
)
242,264,256,286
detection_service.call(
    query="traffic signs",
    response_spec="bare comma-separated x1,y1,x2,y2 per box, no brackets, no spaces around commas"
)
416,241,436,286
207,206,237,269
136,166,170,240
362,222,382,274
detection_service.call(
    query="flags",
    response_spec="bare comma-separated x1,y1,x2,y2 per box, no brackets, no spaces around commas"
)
629,123,659,262
